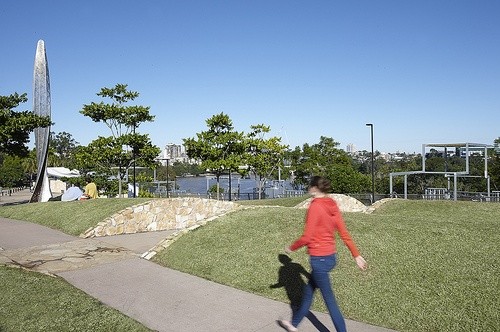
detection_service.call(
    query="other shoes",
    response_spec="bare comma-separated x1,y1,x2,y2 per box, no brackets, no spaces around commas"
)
277,319,298,332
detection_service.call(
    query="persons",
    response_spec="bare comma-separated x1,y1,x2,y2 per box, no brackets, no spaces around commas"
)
278,176,367,332
30,179,36,193
49,182,84,202
84,177,100,199
